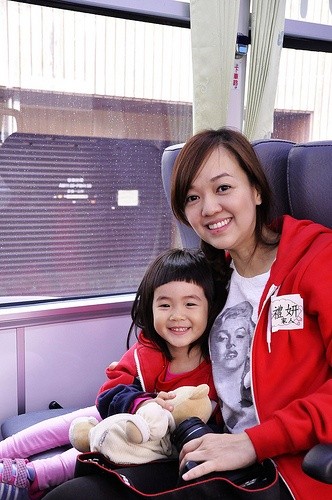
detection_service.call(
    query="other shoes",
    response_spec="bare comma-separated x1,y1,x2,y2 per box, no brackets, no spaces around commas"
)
0,458,36,490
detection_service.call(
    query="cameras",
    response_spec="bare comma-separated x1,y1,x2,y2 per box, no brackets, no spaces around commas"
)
170,417,251,488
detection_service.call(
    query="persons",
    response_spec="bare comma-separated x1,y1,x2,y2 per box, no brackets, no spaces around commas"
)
42,127,332,500
207,302,257,431
1,247,214,500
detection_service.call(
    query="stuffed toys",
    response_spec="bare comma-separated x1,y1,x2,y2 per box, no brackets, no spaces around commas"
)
67,382,212,464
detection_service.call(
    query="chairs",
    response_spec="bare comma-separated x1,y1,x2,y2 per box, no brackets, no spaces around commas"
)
0,139,332,484
